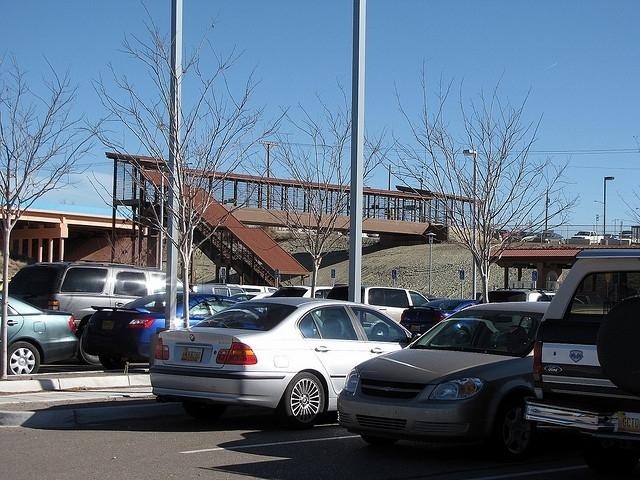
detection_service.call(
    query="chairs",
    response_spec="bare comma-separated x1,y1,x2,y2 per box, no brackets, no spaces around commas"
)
319,317,347,339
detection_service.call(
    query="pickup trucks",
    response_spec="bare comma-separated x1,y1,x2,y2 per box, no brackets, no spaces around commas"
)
572,230,605,246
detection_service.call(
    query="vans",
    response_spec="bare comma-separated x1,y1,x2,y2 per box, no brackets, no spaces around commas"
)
8,261,195,366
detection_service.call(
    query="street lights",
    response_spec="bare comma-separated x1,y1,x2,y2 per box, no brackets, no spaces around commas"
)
426,232,436,296
464,149,477,299
603,176,615,240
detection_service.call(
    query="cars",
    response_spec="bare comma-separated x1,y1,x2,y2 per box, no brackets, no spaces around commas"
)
500,230,564,244
0,292,79,375
619,231,632,239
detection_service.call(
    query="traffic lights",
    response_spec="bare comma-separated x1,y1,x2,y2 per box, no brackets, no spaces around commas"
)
596,214,599,221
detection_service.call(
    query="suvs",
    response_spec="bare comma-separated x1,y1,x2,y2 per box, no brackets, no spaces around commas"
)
522,249,640,444
